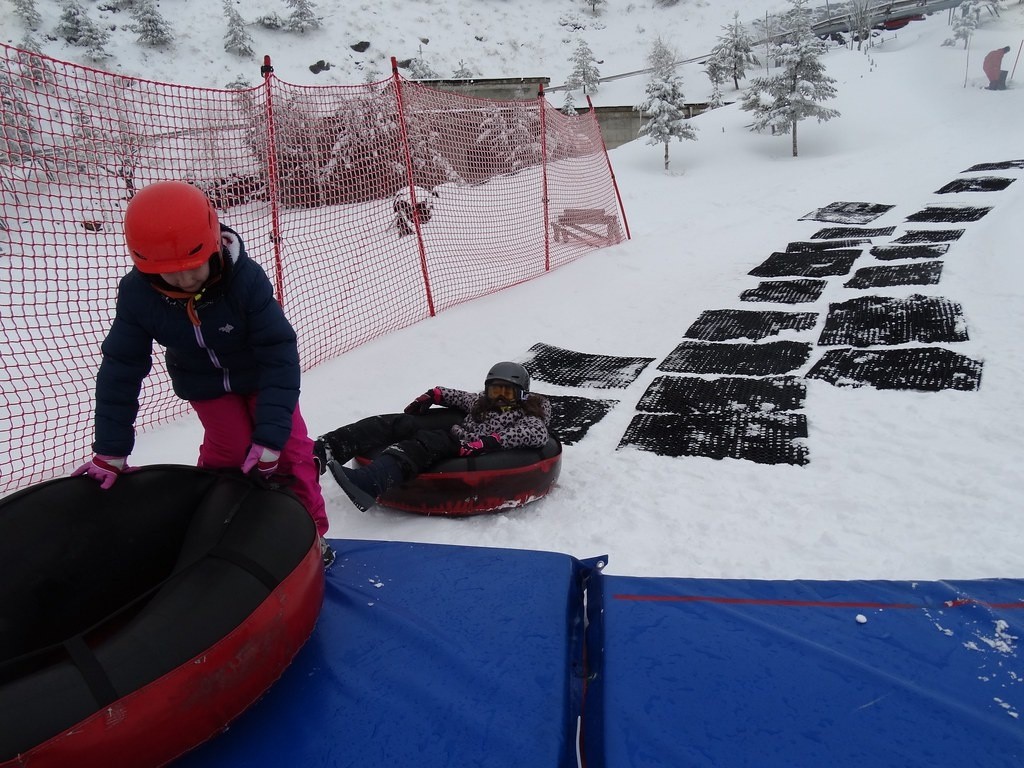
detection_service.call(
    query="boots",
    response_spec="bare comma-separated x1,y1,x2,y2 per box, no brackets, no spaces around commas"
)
326,448,418,512
313,427,354,475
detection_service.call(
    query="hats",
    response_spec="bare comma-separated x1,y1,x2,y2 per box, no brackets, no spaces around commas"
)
1005,46,1010,52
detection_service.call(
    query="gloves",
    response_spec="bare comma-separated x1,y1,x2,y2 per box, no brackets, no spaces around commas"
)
241,443,281,480
71,454,141,489
405,388,441,415
460,432,501,456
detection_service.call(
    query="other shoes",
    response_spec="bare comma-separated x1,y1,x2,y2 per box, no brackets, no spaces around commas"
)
320,538,336,569
989,81,999,90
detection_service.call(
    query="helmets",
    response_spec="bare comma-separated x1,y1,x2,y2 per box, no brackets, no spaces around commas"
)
485,362,530,406
125,181,221,273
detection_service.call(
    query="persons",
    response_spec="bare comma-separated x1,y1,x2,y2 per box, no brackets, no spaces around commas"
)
70,182,336,573
315,362,554,512
983,46,1010,91
